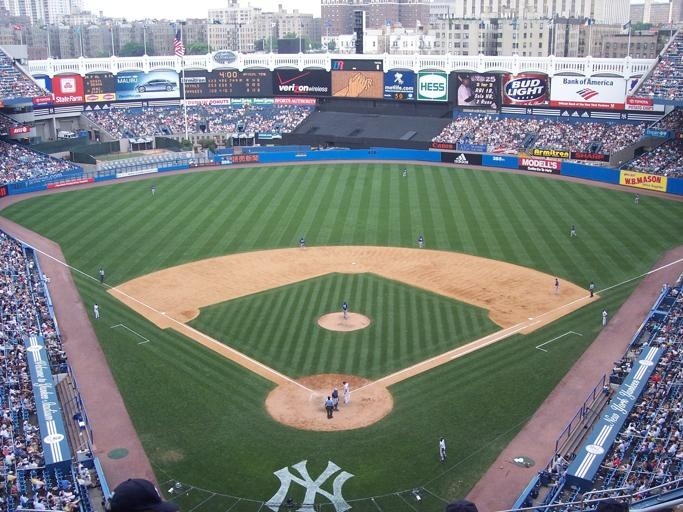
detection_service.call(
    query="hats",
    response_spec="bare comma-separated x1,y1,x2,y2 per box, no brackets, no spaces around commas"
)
445,499,478,512
108,478,179,512
